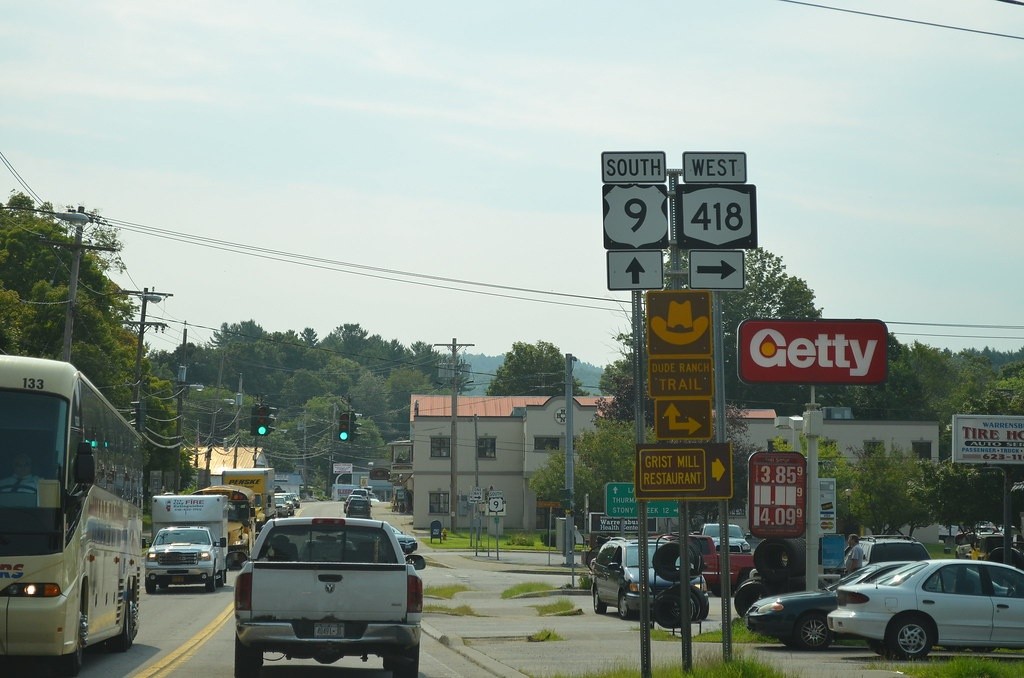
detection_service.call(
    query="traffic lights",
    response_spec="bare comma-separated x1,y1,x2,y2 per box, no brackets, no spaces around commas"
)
130,401,139,430
250,405,277,437
338,412,362,443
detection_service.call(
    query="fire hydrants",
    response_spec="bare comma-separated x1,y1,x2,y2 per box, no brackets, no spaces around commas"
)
442,528,448,540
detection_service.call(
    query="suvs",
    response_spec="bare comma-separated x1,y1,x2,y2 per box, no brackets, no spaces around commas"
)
590,535,712,617
954,531,1004,561
856,534,931,566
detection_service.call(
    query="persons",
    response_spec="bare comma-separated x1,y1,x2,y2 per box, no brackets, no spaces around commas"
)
270,534,292,561
844,533,863,572
0,452,43,493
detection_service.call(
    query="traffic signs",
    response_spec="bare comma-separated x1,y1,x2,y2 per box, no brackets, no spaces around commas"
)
472,488,482,500
647,291,713,438
635,441,733,499
604,481,679,519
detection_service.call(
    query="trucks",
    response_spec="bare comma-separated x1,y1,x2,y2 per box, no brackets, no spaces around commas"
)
222,468,275,526
143,493,229,591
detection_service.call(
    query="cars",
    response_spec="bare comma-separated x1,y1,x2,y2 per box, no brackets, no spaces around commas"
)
826,557,1024,659
274,492,301,516
745,558,1014,651
338,526,418,554
693,522,753,554
343,487,379,519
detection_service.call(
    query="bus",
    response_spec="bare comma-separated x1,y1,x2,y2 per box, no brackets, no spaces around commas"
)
0,352,147,666
193,485,256,563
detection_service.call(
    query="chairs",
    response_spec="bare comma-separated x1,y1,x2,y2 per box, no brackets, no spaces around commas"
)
272,543,299,562
944,571,955,589
959,578,976,595
348,542,376,562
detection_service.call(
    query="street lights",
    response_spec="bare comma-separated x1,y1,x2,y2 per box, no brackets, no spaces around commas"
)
174,398,235,495
845,488,853,527
774,416,803,452
132,384,204,430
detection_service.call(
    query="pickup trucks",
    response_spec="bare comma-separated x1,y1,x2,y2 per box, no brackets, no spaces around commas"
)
663,532,756,588
224,516,426,678
